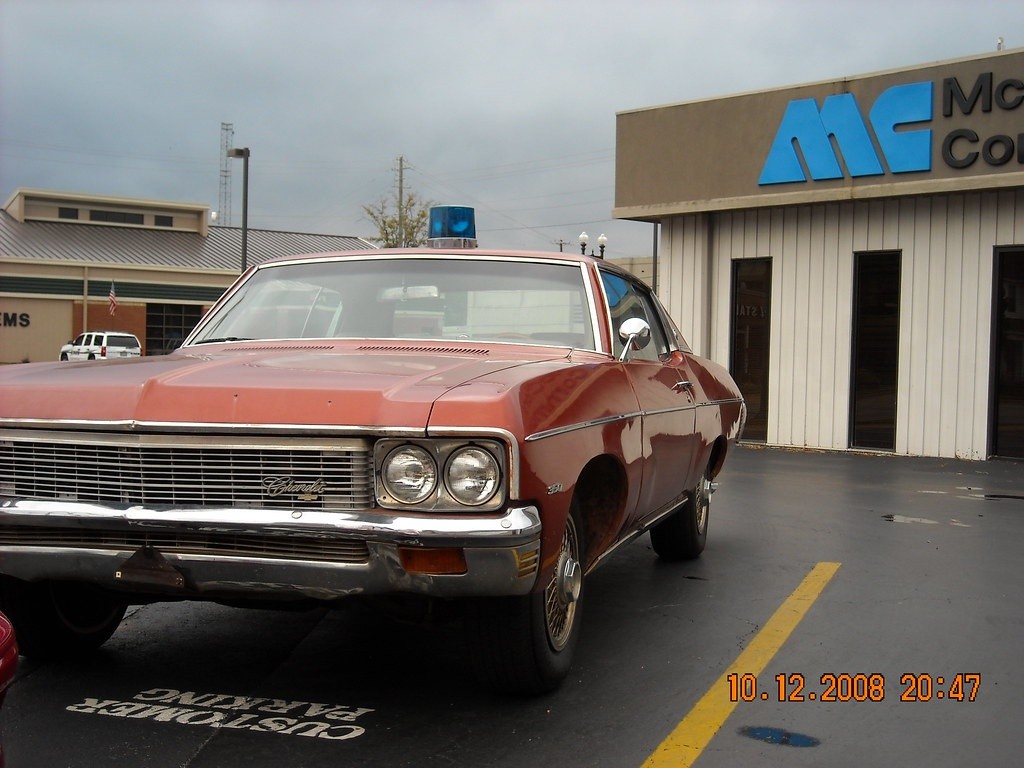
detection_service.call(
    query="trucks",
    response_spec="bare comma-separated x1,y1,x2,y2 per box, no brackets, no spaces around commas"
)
384,283,585,348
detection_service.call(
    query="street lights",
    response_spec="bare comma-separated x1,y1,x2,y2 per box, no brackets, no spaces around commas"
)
227,147,250,274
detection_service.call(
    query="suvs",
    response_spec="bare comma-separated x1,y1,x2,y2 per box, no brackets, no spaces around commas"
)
59,330,141,362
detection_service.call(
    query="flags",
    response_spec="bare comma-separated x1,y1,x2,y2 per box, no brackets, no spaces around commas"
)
109,282,118,317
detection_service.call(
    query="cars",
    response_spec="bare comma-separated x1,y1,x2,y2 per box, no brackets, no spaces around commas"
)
0,248,748,694
